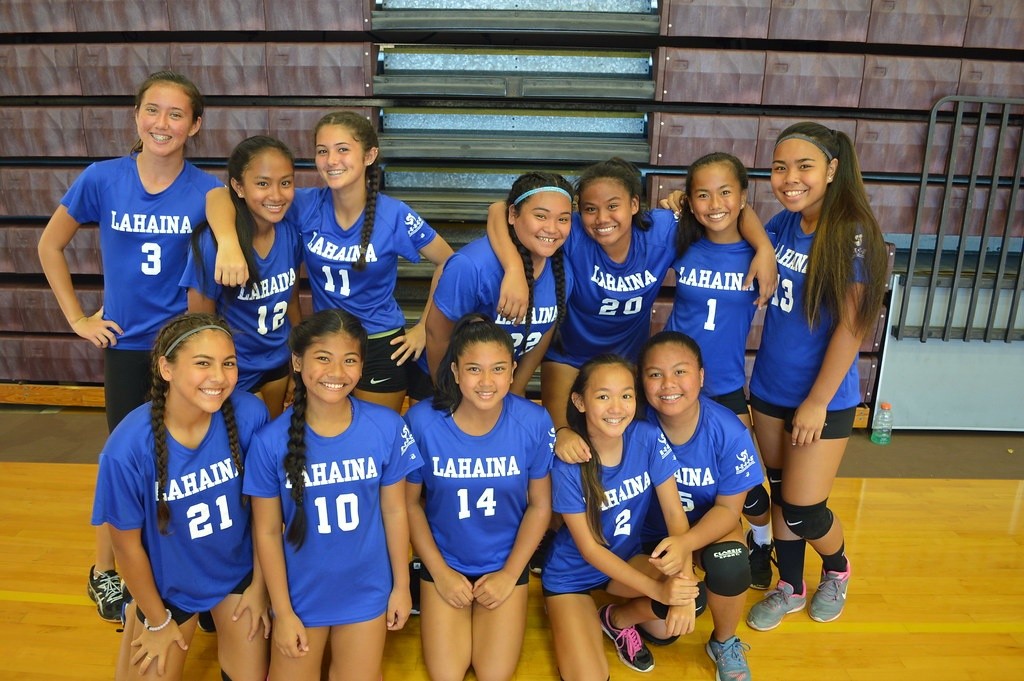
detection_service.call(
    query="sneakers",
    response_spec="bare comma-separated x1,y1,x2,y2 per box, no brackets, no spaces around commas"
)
197,610,215,632
744,526,779,591
597,603,655,672
809,556,850,623
746,579,808,631
408,559,424,615
86,564,123,624
528,528,557,573
706,632,752,681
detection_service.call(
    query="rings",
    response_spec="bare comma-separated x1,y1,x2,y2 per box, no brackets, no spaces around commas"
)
146,656,152,660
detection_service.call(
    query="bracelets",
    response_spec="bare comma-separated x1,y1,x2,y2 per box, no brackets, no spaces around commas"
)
144,609,172,631
556,427,567,436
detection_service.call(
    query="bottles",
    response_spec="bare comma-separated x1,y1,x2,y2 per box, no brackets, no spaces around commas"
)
870,403,892,446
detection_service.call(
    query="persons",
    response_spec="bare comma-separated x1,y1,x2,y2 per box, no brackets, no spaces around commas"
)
178,111,779,614
91,314,271,681
402,316,557,681
542,352,699,681
659,121,888,631
38,71,225,623
555,332,767,681
242,309,424,681
